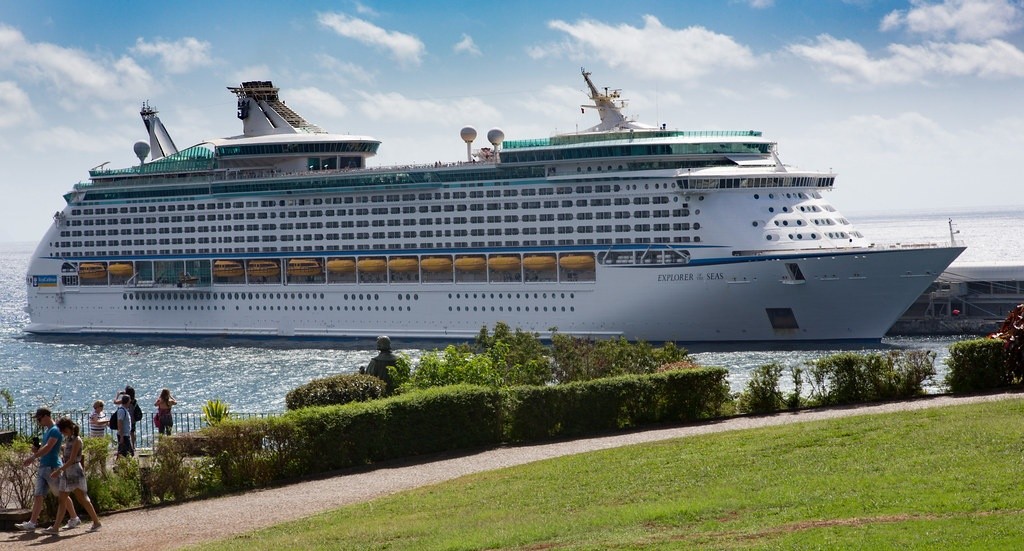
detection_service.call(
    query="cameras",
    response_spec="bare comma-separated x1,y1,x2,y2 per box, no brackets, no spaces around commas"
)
120,391,124,394
33,436,39,447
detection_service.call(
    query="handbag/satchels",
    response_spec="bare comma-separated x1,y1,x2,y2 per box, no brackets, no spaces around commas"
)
154,413,160,428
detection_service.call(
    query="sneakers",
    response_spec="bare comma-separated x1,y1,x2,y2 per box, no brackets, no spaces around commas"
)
84,522,102,532
62,518,81,529
41,526,58,535
14,521,36,533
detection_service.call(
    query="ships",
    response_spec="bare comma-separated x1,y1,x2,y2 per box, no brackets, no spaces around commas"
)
22,66,968,350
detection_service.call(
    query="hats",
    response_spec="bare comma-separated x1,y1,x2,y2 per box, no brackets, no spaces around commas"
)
31,408,51,418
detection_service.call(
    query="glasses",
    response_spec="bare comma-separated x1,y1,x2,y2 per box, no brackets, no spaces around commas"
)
37,417,43,422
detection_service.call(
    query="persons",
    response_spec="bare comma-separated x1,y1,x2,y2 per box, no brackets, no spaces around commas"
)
90,400,111,479
114,386,138,451
14,408,81,532
116,394,135,460
41,417,102,535
366,336,401,376
153,389,177,445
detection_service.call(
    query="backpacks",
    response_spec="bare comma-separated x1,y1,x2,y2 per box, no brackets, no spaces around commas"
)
109,407,126,430
129,400,142,422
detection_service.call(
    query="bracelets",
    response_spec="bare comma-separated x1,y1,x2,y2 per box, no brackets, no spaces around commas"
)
31,455,35,460
59,469,64,474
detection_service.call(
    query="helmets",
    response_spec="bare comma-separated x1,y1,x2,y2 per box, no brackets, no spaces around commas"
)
376,335,392,350
